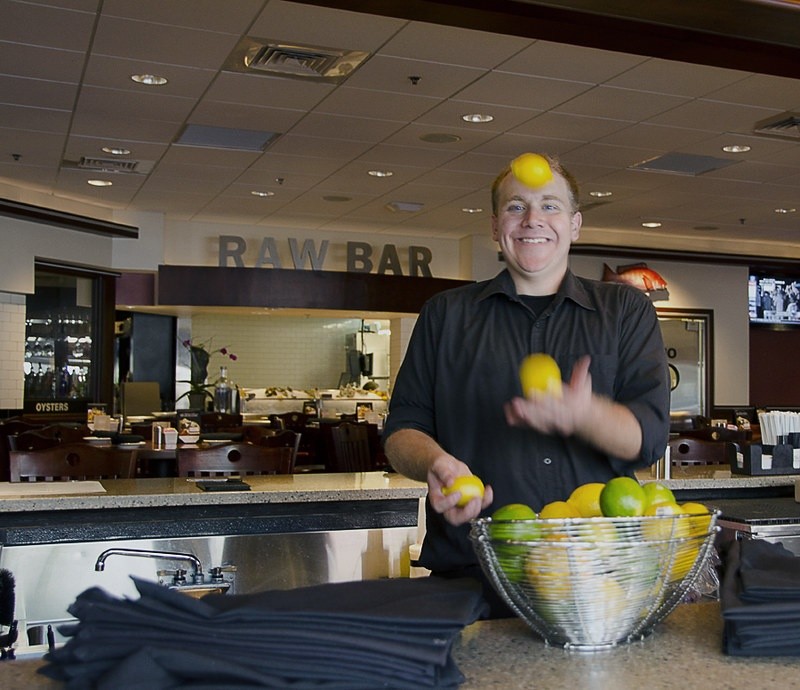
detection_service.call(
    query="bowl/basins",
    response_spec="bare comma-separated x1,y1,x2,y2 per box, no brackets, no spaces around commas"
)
179,443,199,449
179,436,199,443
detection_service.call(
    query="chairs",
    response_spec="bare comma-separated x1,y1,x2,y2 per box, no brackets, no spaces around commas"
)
0,411,384,483
666,415,752,467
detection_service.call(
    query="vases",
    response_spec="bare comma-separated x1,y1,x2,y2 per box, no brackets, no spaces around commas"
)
188,394,207,410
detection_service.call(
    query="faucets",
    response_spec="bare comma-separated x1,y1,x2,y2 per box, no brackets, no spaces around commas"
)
95,548,204,584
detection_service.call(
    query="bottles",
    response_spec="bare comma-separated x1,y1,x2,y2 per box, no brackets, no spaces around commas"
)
154,426,163,444
26,366,87,399
213,366,231,413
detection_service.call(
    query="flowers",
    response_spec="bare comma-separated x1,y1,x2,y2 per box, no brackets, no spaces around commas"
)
175,337,237,402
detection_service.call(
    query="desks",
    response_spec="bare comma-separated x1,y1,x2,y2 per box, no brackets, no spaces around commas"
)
76,437,236,477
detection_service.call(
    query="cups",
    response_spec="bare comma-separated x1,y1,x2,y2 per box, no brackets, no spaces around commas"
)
788,432,800,449
776,434,788,445
718,422,721,427
723,422,726,428
650,443,672,479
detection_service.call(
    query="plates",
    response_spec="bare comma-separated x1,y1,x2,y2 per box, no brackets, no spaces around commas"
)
120,441,146,448
152,411,177,417
83,436,111,441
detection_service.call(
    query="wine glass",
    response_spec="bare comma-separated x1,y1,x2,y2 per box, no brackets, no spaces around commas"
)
24,324,83,358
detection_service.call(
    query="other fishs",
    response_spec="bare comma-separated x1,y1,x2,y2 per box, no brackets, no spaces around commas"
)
600,262,668,291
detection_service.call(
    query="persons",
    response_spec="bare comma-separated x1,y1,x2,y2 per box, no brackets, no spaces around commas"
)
380,151,671,620
762,281,800,321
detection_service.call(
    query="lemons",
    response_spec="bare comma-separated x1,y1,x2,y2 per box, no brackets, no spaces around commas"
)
442,473,485,507
484,476,712,616
510,153,554,187
521,353,565,402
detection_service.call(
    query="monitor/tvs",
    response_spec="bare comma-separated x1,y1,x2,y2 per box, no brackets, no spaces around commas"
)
748,272,800,325
